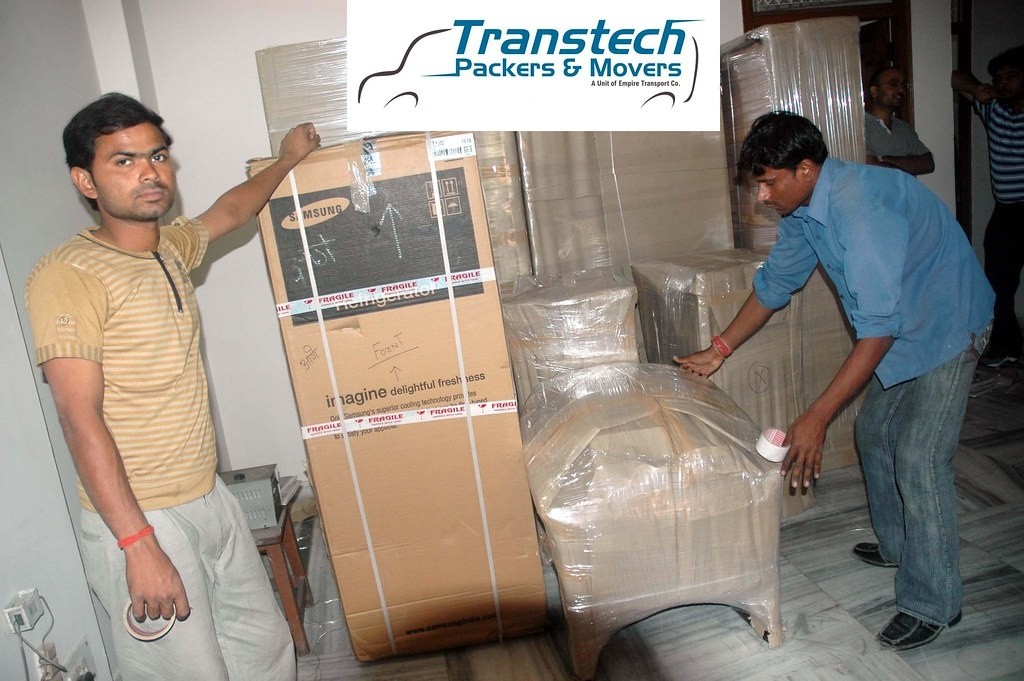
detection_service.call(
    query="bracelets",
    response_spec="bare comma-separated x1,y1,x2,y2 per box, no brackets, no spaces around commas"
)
118,525,154,550
711,337,733,360
877,155,883,162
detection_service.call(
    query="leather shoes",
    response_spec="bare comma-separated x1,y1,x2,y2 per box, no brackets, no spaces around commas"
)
853,543,900,568
875,607,963,652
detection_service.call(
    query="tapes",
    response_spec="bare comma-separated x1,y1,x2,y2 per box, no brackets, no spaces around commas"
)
755,427,792,464
122,598,177,641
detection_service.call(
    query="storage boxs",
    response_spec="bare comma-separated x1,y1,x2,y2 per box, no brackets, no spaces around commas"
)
217,463,283,529
245,16,872,680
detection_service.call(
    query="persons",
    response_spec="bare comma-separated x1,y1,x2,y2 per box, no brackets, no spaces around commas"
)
670,112,998,652
26,92,322,681
863,69,936,177
951,46,1024,368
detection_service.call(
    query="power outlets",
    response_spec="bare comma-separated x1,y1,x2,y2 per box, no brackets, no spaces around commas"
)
67,657,89,681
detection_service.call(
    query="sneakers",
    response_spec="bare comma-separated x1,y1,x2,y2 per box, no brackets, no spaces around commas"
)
981,346,1021,368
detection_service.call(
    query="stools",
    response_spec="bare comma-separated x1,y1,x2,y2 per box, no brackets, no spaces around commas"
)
249,505,315,657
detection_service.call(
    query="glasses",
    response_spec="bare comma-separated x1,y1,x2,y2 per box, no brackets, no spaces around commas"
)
876,79,911,90
